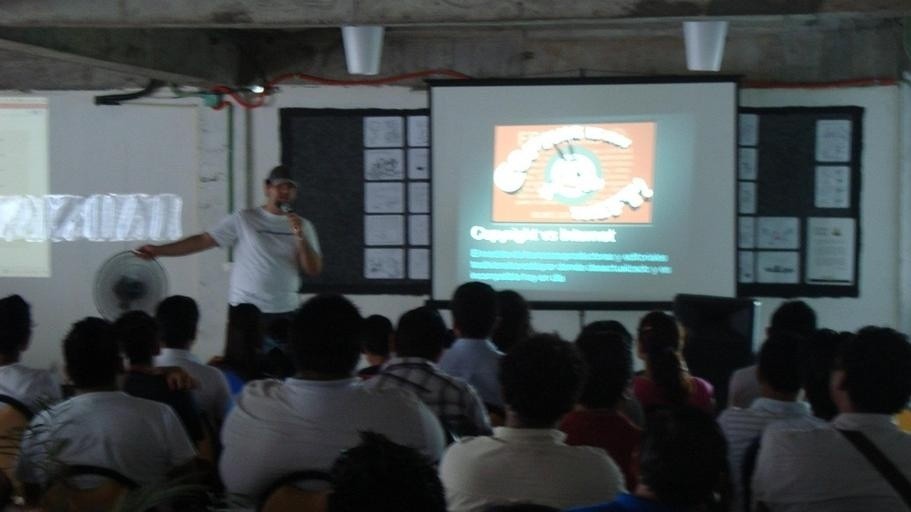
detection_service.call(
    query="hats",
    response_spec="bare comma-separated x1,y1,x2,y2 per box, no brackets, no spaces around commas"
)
636,308,683,346
265,164,301,189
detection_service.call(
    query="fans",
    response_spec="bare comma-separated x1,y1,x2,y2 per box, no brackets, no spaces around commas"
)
91,250,169,319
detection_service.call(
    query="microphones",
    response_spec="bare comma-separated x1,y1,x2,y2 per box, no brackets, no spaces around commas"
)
279,204,295,212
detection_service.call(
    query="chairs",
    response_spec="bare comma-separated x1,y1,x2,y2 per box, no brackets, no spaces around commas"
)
1,393,36,499
256,470,337,512
31,464,140,512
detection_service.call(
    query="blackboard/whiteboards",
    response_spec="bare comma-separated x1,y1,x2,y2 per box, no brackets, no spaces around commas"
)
277,104,864,300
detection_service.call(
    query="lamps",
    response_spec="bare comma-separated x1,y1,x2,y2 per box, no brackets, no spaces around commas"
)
681,20,731,75
340,21,387,78
242,73,275,99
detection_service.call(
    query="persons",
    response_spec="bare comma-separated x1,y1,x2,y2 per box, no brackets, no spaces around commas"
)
134,166,323,382
